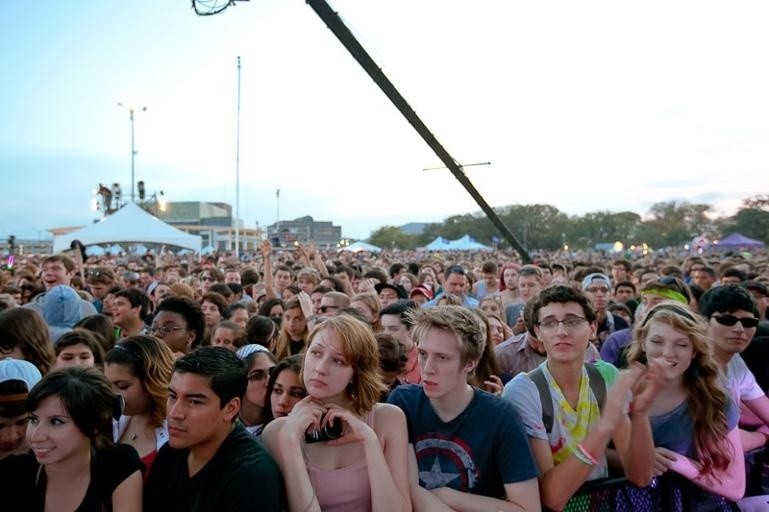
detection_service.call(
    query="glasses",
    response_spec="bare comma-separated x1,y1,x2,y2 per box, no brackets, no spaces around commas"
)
706,312,762,332
532,315,589,330
317,305,341,313
585,286,610,294
86,269,114,281
200,275,218,283
609,266,628,270
478,294,503,304
247,369,276,385
149,324,190,336
616,289,633,295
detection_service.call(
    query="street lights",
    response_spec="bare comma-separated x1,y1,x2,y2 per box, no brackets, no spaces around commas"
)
117,103,148,203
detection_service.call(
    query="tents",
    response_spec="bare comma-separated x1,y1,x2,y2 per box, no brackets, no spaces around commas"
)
340,234,493,250
53,202,200,257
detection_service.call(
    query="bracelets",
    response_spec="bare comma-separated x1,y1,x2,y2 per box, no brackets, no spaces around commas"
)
575,442,600,466
572,449,592,468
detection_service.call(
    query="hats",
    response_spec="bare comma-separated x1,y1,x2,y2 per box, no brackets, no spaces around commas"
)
0,354,43,403
409,284,432,301
582,272,612,293
373,281,409,299
551,262,566,273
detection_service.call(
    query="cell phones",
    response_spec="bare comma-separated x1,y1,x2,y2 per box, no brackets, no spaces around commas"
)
304,413,345,444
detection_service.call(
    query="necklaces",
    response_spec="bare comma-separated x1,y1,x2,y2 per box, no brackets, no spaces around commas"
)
129,416,155,440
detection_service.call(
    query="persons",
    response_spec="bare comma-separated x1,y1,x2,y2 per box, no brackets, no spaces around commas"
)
349,292,381,325
276,294,317,357
110,290,152,340
198,293,227,323
0,240,768,289
372,334,411,404
150,296,206,357
0,293,20,312
465,309,511,397
101,334,177,484
21,284,35,304
605,297,745,512
318,292,349,314
2,358,46,458
2,308,56,376
492,294,600,376
279,287,300,305
9,290,21,304
72,316,116,353
381,299,426,384
101,286,120,315
373,281,408,308
385,300,540,511
231,313,280,355
228,282,242,307
197,274,408,285
2,365,147,511
260,314,415,511
51,327,105,371
147,281,170,312
501,284,664,511
699,284,768,452
21,284,97,344
310,289,335,317
146,347,289,510
244,353,308,443
234,344,277,426
260,298,285,320
209,321,242,352
91,328,110,355
600,276,692,366
224,305,249,332
172,285,194,302
207,286,231,308
239,297,259,317
